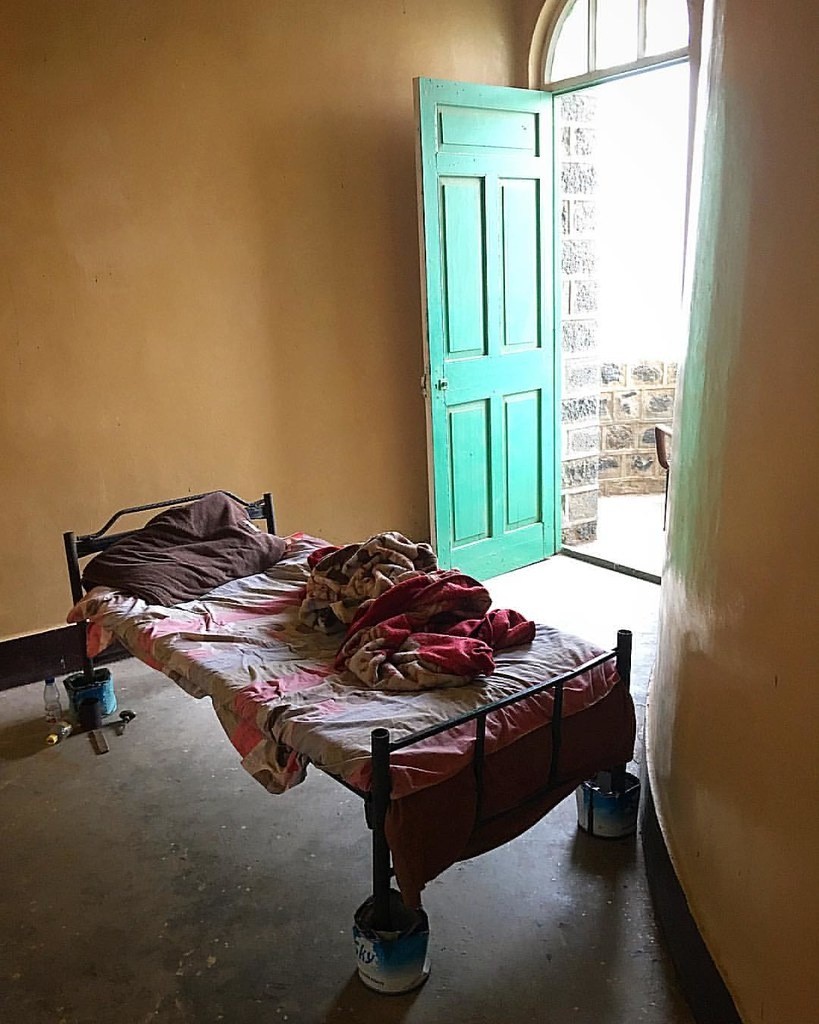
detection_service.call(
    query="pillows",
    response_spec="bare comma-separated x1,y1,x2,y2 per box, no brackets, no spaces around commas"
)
81,491,288,608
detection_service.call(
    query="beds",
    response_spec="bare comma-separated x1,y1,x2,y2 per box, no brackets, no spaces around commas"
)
62,487,640,994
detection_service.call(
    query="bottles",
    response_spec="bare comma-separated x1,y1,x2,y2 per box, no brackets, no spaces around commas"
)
44,676,63,722
46,715,75,745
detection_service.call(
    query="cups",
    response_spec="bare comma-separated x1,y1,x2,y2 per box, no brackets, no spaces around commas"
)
80,697,102,730
